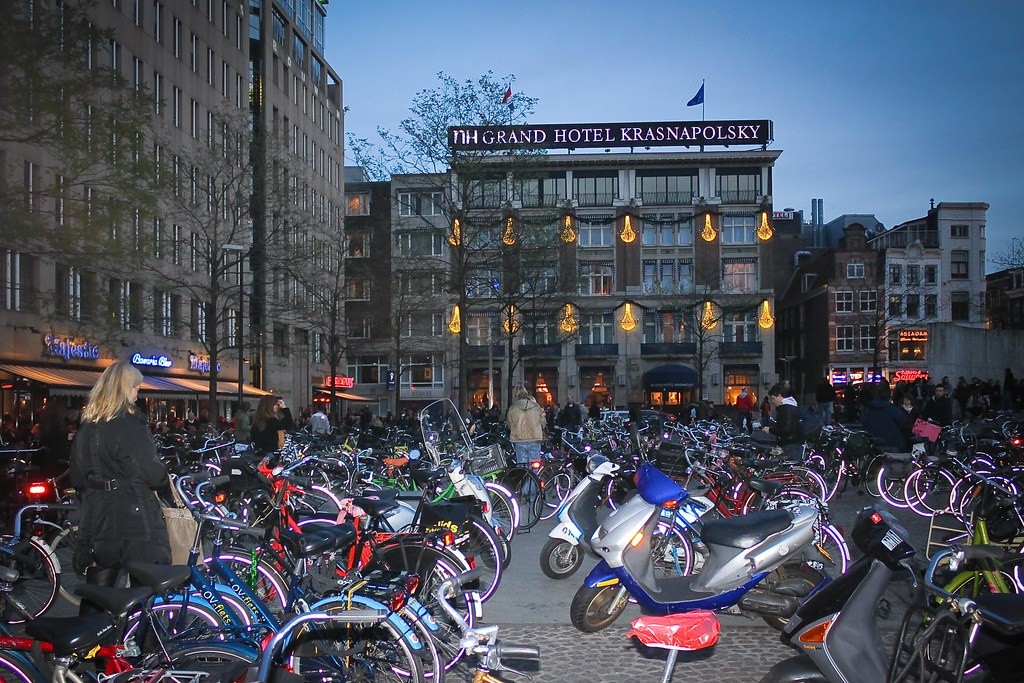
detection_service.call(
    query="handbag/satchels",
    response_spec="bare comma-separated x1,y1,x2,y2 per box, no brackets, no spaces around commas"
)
151,471,204,565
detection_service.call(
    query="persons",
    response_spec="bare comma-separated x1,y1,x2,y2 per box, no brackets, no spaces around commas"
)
345,405,430,434
232,394,331,450
545,394,600,445
844,367,1024,478
67,359,171,624
736,386,754,436
0,402,209,476
507,385,546,504
759,383,822,459
469,403,500,424
814,376,837,424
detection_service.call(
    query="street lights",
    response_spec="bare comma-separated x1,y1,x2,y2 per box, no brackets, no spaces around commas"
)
223,244,244,407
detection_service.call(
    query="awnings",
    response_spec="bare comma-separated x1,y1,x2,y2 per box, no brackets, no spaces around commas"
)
642,364,698,390
0,363,273,402
317,389,376,402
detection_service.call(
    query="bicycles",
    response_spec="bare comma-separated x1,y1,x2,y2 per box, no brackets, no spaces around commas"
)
0,398,720,683
836,410,1024,539
502,409,850,577
887,547,1024,683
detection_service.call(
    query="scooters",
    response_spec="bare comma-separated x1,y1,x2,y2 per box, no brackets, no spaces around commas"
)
539,445,835,632
759,499,1024,683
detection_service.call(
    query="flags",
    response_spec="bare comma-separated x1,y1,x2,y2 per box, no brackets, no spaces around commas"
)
501,86,511,104
687,83,703,106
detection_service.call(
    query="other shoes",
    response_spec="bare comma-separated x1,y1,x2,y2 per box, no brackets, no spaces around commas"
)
749,431,754,436
